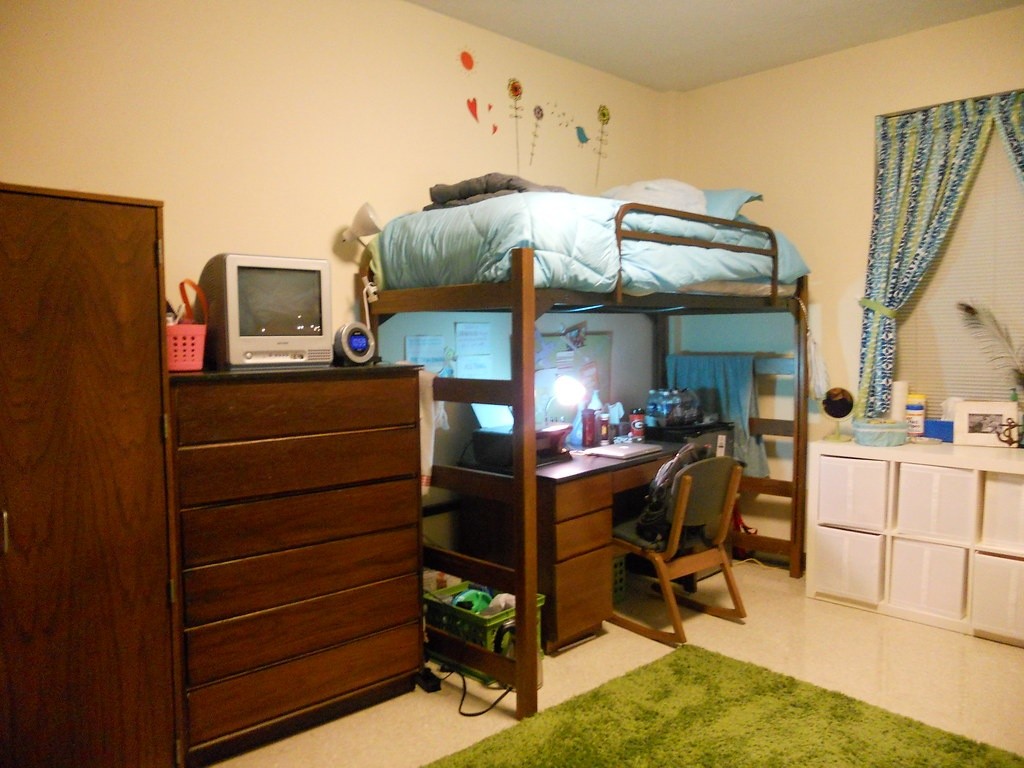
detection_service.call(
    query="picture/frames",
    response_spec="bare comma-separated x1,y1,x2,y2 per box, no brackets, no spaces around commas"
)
953,402,1019,448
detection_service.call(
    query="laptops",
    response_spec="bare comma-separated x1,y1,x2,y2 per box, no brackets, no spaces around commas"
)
584,443,662,460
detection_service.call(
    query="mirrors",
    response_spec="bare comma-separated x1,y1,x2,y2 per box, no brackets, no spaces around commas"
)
818,387,858,442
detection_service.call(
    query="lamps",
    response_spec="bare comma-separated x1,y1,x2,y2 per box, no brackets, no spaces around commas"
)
586,383,603,411
544,374,592,418
341,201,384,303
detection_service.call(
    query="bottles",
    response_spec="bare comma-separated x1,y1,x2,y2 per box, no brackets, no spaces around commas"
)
905,393,925,437
581,388,701,446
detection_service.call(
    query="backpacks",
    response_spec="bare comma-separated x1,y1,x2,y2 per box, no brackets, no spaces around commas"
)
635,443,713,539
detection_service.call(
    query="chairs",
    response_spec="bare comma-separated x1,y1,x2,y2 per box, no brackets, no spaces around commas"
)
613,456,747,644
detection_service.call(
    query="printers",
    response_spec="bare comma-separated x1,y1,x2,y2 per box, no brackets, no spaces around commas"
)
473,422,572,473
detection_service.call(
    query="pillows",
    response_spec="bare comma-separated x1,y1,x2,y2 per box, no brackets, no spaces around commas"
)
703,189,764,220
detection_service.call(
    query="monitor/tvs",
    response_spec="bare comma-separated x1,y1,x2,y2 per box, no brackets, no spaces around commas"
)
190,252,334,369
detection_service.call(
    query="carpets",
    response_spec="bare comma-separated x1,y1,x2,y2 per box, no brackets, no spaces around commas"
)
423,646,1024,768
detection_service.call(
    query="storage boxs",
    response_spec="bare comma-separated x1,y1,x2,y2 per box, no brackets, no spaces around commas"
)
422,581,546,686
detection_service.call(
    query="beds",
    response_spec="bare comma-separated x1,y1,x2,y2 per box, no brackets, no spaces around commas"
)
359,204,810,314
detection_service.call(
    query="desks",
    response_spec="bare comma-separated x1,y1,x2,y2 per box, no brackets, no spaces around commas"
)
458,444,676,654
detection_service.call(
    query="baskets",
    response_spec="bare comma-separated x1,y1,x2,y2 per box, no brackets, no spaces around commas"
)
167,279,208,372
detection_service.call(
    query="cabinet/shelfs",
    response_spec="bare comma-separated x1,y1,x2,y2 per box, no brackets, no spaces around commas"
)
806,440,1023,649
167,364,425,767
